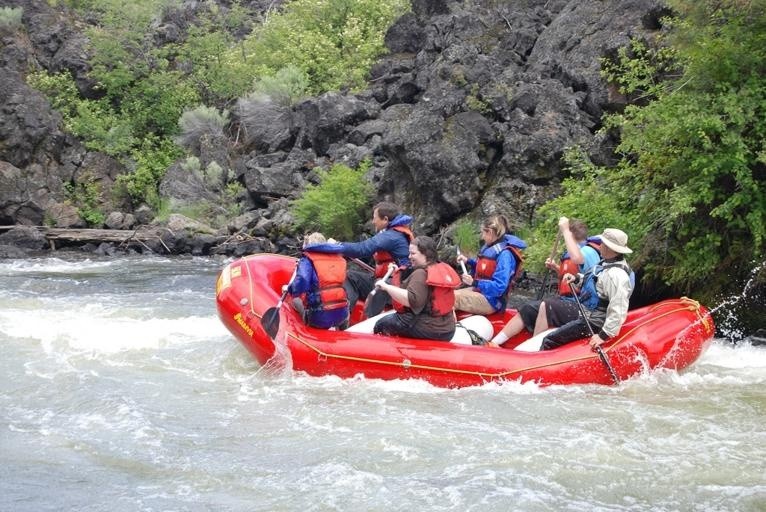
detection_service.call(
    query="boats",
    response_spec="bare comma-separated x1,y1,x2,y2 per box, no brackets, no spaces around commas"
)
215,244,715,390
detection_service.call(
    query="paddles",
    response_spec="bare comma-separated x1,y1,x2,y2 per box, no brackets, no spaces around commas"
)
362,260,395,317
260,259,298,339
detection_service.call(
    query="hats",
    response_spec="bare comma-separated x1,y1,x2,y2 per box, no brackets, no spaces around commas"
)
595,227,633,255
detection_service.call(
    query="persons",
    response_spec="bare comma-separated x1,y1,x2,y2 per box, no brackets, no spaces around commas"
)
327,201,415,322
492,216,603,347
281,232,349,331
538,227,632,351
374,236,456,342
453,216,527,316
